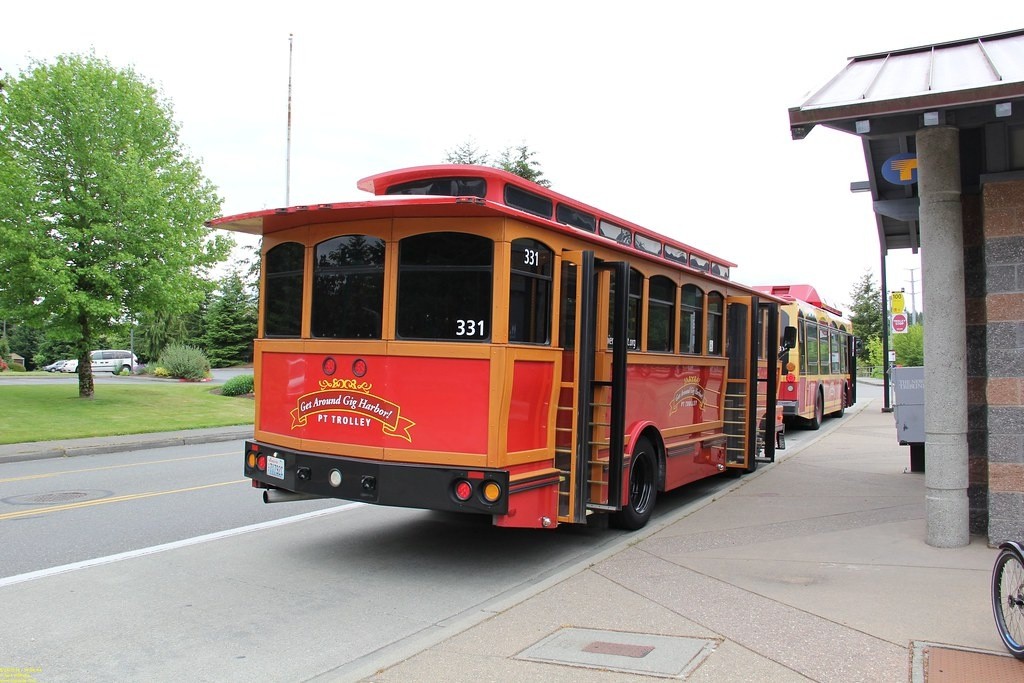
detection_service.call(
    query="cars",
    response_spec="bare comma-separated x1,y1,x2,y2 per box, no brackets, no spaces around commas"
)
44,360,78,372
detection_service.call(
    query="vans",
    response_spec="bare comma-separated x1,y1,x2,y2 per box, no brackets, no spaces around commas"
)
67,350,138,375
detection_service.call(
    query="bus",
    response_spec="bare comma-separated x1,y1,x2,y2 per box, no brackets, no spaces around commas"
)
203,165,796,531
751,284,856,430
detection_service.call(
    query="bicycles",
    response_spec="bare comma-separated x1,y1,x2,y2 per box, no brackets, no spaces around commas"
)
991,542,1024,659
